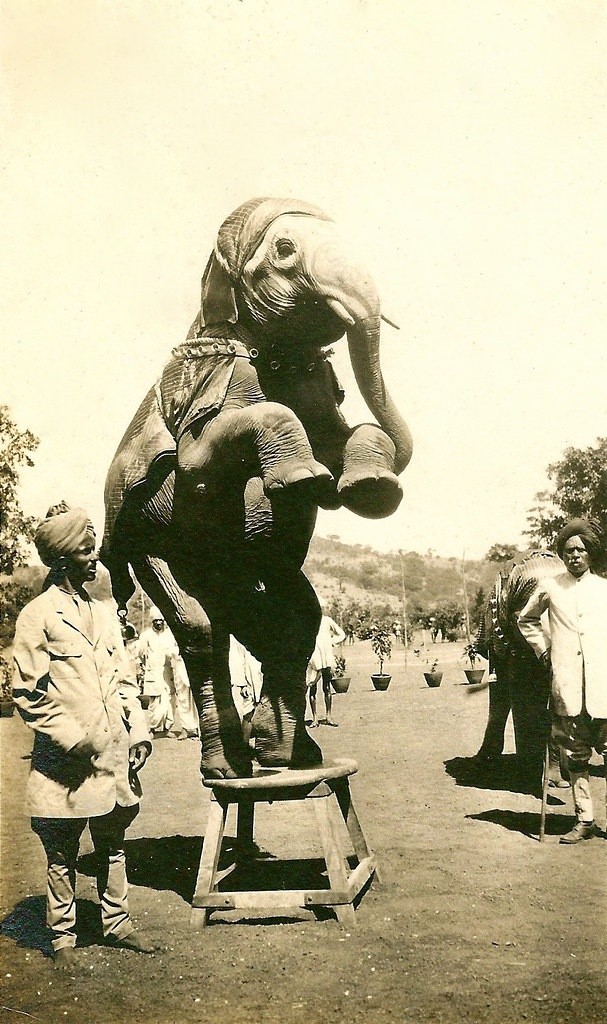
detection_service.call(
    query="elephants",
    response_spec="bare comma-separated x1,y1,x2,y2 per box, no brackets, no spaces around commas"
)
97,196,413,781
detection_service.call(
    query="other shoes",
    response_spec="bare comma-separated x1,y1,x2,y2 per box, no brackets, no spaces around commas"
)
559,821,595,844
177,730,188,740
149,731,156,740
164,731,176,738
325,720,339,727
309,721,319,728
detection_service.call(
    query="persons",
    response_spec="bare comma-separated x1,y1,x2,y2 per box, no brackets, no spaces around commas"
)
516,518,607,845
121,601,346,742
12,498,159,973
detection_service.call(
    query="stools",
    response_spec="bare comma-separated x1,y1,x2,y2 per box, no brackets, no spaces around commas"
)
187,758,382,929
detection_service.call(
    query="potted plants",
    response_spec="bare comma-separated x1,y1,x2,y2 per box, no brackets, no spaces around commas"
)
369,620,392,690
413,642,444,687
460,645,485,684
0,654,16,717
331,654,351,693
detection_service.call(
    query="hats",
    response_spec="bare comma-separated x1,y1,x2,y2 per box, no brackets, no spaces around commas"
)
149,605,165,622
34,501,96,567
556,518,606,560
